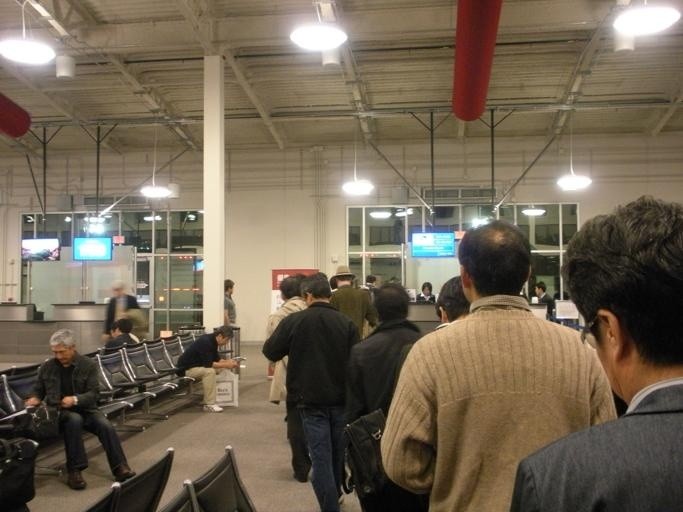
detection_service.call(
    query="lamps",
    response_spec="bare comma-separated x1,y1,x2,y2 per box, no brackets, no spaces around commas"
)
137,117,174,198
554,105,593,195
0,0,57,68
338,114,376,199
609,1,682,42
287,1,350,54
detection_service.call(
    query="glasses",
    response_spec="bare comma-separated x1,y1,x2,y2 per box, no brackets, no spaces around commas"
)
580,314,599,351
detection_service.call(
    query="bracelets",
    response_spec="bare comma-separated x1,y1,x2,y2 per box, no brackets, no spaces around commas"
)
72,394,81,407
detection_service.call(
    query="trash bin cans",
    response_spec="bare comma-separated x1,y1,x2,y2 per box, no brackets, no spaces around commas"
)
178,326,204,335
213,327,241,381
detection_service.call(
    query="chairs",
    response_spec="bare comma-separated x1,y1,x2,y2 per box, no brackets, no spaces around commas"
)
0,331,235,477
114,446,175,511
182,442,257,512
78,482,114,511
158,479,200,511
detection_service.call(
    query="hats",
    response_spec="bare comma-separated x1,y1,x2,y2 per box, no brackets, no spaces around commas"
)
332,265,353,278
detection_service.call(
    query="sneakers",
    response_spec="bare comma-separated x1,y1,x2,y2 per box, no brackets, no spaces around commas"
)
203,404,224,412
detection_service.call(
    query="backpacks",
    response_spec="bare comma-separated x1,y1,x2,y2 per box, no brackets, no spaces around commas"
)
340,343,430,512
0,438,39,502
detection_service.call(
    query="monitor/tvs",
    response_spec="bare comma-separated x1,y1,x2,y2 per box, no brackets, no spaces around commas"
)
73,237,112,261
22,238,60,260
411,232,455,257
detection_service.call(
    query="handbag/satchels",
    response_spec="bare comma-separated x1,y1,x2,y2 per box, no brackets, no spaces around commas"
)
0,401,59,437
216,368,239,406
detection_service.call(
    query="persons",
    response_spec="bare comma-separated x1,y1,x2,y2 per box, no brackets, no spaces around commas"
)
100,318,142,356
23,327,135,488
380,218,618,511
393,275,471,393
267,275,309,482
362,274,377,303
262,273,362,512
512,195,683,511
346,282,429,510
329,266,377,336
223,279,237,325
531,281,554,313
413,281,436,302
102,279,147,341
175,324,240,413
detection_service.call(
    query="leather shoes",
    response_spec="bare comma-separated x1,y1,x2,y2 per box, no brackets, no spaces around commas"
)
114,464,136,481
68,471,84,489
